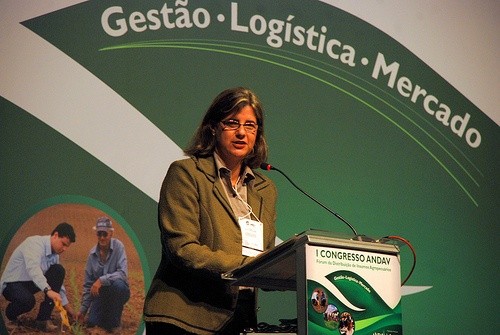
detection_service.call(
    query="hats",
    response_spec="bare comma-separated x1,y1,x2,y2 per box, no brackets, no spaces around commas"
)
93,217,115,231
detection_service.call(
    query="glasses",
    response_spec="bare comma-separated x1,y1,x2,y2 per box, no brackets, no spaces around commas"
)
221,120,258,131
97,233,108,237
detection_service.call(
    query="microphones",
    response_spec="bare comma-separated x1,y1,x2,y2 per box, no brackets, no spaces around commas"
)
260,162,357,235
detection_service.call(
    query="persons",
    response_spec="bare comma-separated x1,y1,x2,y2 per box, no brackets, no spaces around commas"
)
0,222,77,330
311,290,355,335
142,86,278,335
77,217,131,335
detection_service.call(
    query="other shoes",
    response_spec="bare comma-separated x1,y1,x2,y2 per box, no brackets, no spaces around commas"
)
34,320,57,331
1,308,18,329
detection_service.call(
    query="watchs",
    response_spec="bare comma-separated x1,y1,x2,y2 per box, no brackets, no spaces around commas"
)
44,287,51,293
